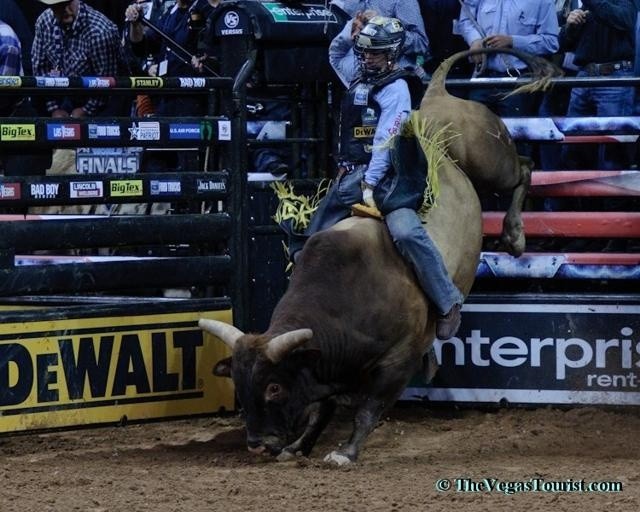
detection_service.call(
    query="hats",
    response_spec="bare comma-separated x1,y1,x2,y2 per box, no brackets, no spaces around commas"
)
353,16,406,80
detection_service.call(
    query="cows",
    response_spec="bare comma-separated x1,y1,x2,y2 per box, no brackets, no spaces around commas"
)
198,46,562,473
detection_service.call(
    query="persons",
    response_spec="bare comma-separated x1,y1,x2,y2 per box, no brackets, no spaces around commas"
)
292,8,465,340
0,2,637,298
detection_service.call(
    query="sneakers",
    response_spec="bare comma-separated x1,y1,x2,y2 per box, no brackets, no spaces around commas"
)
436,304,461,339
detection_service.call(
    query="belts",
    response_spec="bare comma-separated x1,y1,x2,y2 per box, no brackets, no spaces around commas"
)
589,62,631,75
